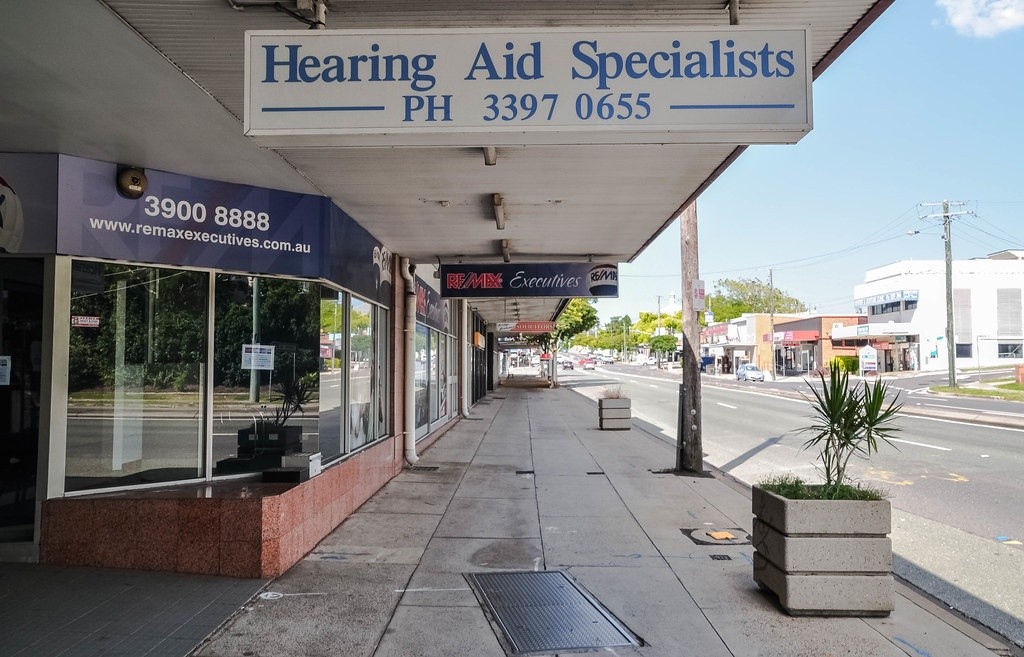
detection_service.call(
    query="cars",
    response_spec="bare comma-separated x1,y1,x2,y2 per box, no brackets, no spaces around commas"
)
562,361,574,370
577,355,614,371
736,364,765,382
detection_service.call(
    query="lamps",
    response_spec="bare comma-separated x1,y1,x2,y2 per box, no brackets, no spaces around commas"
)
502,240,512,263
482,148,501,168
493,193,506,231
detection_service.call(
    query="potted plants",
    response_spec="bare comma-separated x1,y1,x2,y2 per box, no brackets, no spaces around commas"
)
597,381,633,430
235,378,319,463
749,354,909,619
